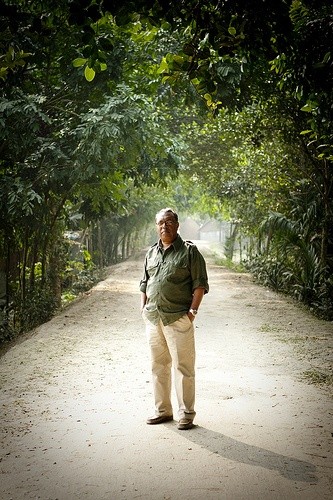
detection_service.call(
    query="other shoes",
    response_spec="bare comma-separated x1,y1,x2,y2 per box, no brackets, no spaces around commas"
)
145,414,173,425
176,417,193,430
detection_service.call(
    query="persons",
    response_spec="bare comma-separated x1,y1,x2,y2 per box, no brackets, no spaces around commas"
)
139,208,209,430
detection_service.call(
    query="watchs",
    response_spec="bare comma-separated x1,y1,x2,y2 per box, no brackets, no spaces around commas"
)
188,309,198,316
